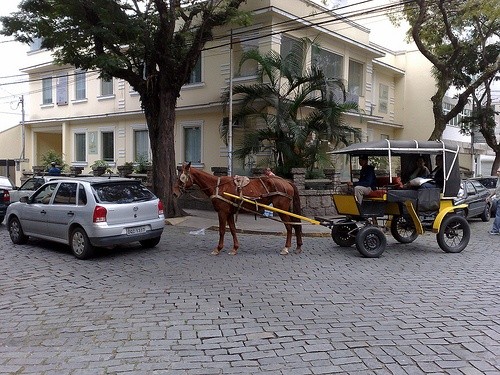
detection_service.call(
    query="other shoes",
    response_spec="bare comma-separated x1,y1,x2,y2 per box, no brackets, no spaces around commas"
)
260,215,268,218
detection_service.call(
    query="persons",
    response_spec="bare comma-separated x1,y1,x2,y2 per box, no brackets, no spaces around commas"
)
420,154,444,189
404,156,430,186
41,185,70,204
48,162,61,176
486,168,500,235
347,155,377,206
263,168,275,217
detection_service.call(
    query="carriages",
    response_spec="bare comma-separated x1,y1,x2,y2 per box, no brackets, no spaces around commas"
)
172,139,472,257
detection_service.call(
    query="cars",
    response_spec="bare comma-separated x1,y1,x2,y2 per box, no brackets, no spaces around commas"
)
441,178,492,221
0,176,14,220
6,176,73,203
468,176,500,214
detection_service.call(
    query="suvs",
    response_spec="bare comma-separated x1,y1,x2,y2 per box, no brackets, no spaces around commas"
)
3,177,167,259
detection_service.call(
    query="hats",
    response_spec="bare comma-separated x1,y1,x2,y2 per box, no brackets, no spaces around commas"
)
358,153,368,159
267,168,271,170
50,162,56,165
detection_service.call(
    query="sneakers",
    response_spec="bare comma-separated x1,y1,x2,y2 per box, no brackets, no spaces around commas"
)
488,229,500,235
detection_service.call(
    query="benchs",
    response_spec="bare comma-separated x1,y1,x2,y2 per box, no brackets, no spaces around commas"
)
342,176,403,201
